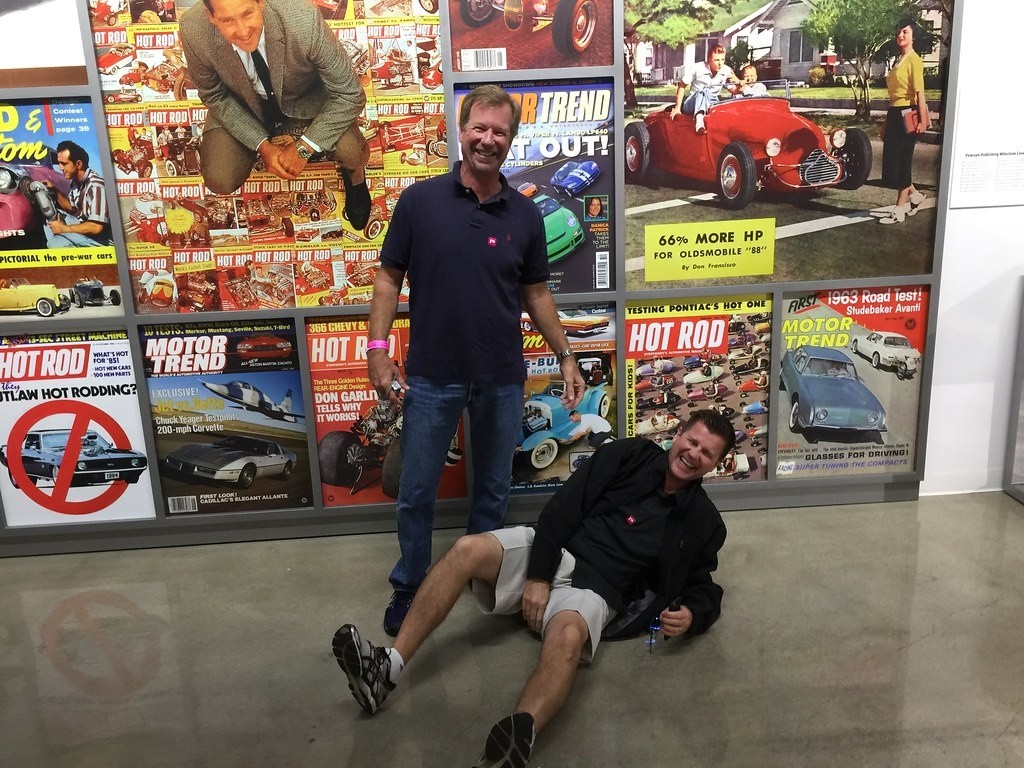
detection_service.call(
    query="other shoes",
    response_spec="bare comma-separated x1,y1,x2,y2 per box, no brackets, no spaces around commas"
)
879,191,926,225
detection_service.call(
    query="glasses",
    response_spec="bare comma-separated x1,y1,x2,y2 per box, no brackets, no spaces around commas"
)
649,617,662,654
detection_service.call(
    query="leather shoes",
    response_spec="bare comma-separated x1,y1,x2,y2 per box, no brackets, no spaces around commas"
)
341,164,371,231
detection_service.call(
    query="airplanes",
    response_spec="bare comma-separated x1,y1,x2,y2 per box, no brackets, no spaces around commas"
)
197,379,306,423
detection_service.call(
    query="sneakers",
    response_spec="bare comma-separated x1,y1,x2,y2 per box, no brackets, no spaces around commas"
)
332,623,397,715
469,711,536,767
383,571,427,637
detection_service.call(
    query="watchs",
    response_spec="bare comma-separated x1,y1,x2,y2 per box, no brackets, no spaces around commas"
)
295,139,312,161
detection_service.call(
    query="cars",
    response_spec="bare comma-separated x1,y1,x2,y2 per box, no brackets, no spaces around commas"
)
519,381,611,473
165,434,298,488
0,277,71,317
521,308,611,337
0,430,148,489
0,145,70,250
780,345,887,435
852,331,921,368
578,357,612,386
236,331,291,362
624,79,873,210
98,43,137,75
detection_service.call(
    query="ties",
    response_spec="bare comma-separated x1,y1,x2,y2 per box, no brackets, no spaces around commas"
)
251,49,281,112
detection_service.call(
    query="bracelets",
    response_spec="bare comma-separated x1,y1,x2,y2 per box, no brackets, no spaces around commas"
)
557,349,575,363
366,340,391,352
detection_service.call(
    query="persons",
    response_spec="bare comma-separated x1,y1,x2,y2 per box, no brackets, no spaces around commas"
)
826,361,855,380
178,0,374,231
633,311,772,482
668,44,748,134
331,407,736,768
587,362,602,385
367,84,589,644
82,275,104,287
44,139,115,251
556,410,613,454
876,19,926,224
731,65,767,100
0,279,22,290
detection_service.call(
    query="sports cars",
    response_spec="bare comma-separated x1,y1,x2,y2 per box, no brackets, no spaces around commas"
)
531,193,586,266
515,181,538,198
549,158,601,198
